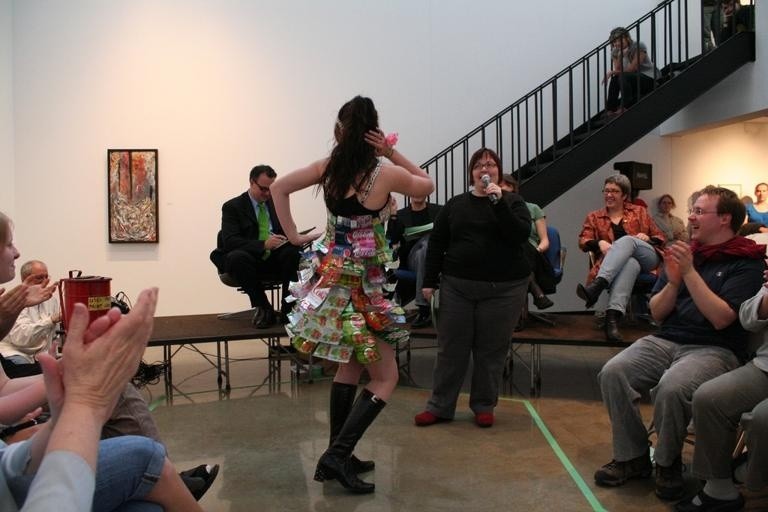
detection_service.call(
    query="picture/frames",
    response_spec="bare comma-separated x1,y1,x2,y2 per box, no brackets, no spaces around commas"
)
107,149,159,244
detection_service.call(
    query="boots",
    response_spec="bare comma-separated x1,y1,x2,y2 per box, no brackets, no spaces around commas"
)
313,381,387,494
577,278,606,309
604,311,623,341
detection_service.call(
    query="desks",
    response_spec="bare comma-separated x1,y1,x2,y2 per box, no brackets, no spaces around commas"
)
71,312,313,403
389,309,661,398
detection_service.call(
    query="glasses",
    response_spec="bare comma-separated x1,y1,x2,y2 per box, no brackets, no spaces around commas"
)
689,207,717,217
602,188,622,195
254,182,269,193
474,161,496,170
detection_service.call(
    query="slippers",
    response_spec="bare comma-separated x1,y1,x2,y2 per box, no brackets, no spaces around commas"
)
676,486,746,512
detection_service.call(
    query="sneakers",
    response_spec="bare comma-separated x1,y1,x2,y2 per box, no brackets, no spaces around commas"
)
655,462,683,502
533,296,553,309
415,411,438,425
514,319,528,333
477,412,493,427
594,453,652,487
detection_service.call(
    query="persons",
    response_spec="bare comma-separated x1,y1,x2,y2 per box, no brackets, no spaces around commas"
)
498,172,557,310
211,163,305,329
0,214,222,512
268,94,435,495
600,25,657,118
413,145,534,427
702,0,755,53
387,193,445,330
573,172,767,511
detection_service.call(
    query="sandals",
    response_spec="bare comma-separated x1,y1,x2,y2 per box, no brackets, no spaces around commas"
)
180,464,219,501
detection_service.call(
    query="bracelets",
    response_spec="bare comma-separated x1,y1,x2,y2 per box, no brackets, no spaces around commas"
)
382,130,399,159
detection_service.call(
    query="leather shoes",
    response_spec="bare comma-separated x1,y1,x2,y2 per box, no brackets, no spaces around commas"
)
257,311,277,329
410,311,431,328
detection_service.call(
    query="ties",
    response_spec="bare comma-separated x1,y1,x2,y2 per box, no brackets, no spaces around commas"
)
258,204,270,260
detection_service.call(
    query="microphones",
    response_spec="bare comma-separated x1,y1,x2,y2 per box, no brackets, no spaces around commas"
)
481,173,500,206
